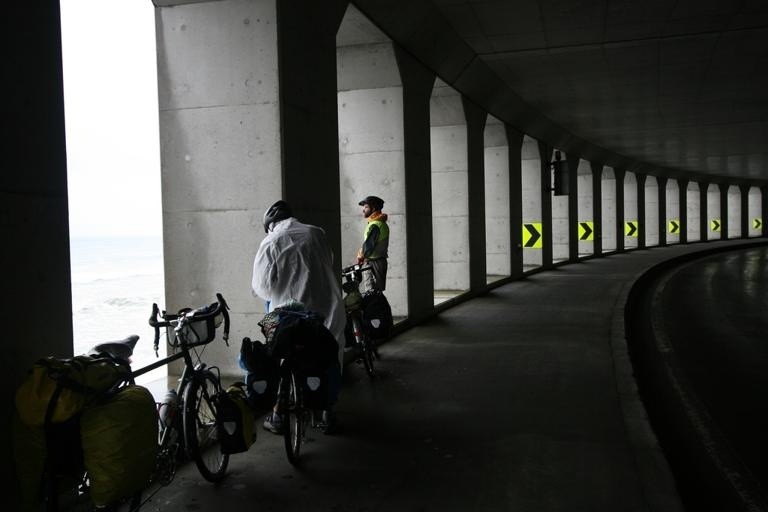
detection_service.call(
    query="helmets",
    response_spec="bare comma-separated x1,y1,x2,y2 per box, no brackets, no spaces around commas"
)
263,200,292,233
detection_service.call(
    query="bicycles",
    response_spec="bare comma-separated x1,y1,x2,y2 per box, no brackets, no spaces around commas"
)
36,293,257,512
274,298,338,466
342,261,382,378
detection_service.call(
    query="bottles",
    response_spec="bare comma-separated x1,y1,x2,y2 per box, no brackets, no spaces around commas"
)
160,389,178,425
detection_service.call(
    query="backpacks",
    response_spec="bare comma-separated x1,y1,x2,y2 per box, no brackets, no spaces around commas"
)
364,288,392,337
11,354,159,508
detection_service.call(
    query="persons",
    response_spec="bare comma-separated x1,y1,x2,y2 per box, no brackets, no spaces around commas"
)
355,196,389,292
252,200,347,435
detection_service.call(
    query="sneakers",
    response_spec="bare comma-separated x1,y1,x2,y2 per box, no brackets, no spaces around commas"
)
264,416,280,434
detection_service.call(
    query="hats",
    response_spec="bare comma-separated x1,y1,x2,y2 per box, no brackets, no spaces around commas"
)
359,196,384,209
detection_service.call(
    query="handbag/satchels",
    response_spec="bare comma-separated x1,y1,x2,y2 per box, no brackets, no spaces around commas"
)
241,312,338,409
210,382,257,454
